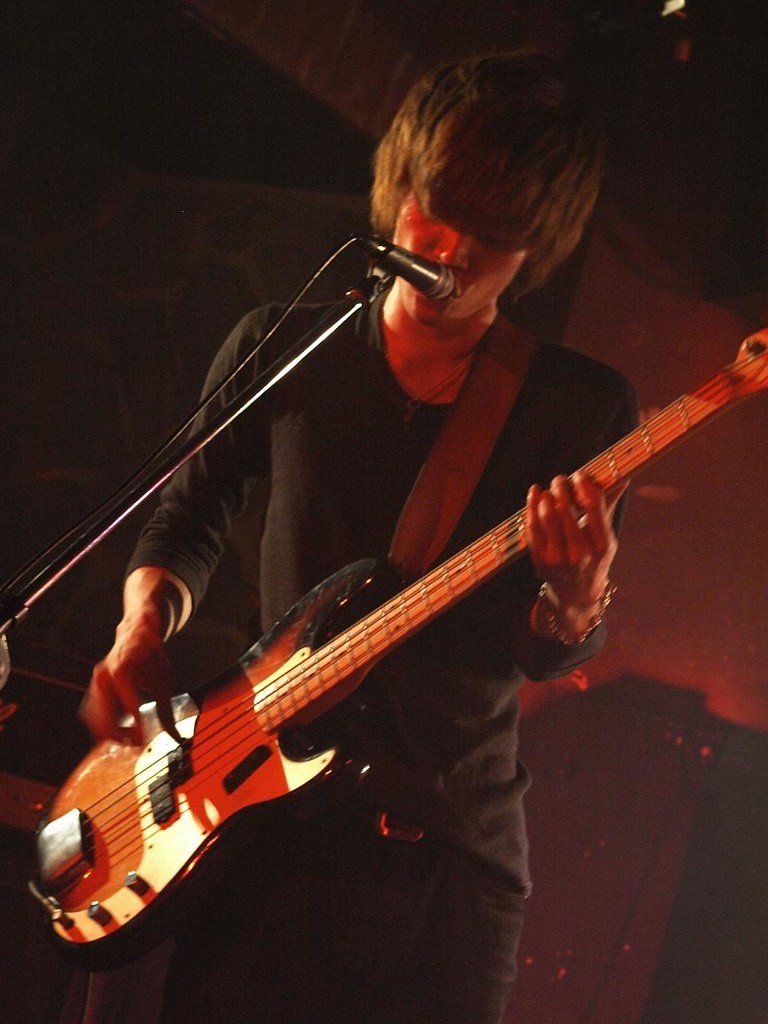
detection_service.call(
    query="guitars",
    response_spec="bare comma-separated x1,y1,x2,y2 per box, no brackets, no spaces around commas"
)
28,324,768,948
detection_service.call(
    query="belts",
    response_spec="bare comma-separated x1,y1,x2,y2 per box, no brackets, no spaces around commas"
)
310,794,425,841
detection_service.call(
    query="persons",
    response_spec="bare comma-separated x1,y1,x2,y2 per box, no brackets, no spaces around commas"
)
78,50,639,1023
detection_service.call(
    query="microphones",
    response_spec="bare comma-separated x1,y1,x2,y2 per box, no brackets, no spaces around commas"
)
353,234,454,299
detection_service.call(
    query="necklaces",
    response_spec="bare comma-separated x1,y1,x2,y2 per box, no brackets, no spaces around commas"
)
404,346,477,423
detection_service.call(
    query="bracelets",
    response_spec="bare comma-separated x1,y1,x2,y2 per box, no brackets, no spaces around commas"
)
538,580,618,646
